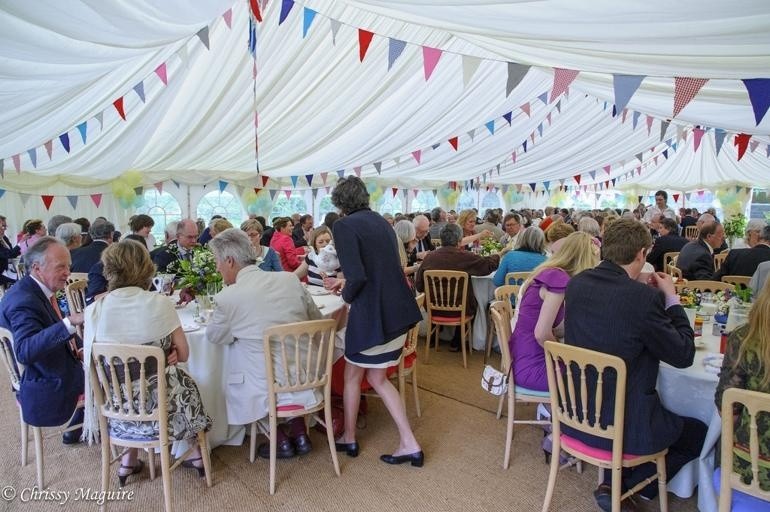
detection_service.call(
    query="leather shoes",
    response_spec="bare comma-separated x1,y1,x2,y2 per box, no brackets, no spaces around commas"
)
594,483,641,512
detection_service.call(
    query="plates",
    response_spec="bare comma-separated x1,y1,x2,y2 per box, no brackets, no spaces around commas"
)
700,351,726,374
310,290,332,296
181,323,201,333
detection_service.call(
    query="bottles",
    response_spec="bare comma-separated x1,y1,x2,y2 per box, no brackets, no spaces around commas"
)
693,311,703,347
696,291,713,322
206,280,217,304
720,333,727,353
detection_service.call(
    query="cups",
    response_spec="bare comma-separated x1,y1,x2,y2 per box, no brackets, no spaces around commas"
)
161,278,172,296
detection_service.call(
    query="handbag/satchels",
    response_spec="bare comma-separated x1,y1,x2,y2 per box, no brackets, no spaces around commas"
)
480,363,509,396
314,394,367,437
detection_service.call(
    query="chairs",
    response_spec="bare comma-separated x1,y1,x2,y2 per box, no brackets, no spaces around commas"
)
1,226,770,512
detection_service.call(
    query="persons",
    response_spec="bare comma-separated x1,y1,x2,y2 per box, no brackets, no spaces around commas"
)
0,237,87,444
715,272,770,492
508,231,599,461
83,238,212,488
321,175,426,468
205,229,325,458
0,191,769,404
564,217,710,512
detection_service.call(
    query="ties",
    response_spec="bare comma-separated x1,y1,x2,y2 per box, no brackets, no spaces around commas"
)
50,294,79,361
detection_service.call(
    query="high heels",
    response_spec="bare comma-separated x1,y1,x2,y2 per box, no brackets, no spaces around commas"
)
537,404,572,466
62,418,423,486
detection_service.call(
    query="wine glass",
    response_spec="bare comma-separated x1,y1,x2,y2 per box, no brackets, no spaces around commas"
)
488,230,496,245
